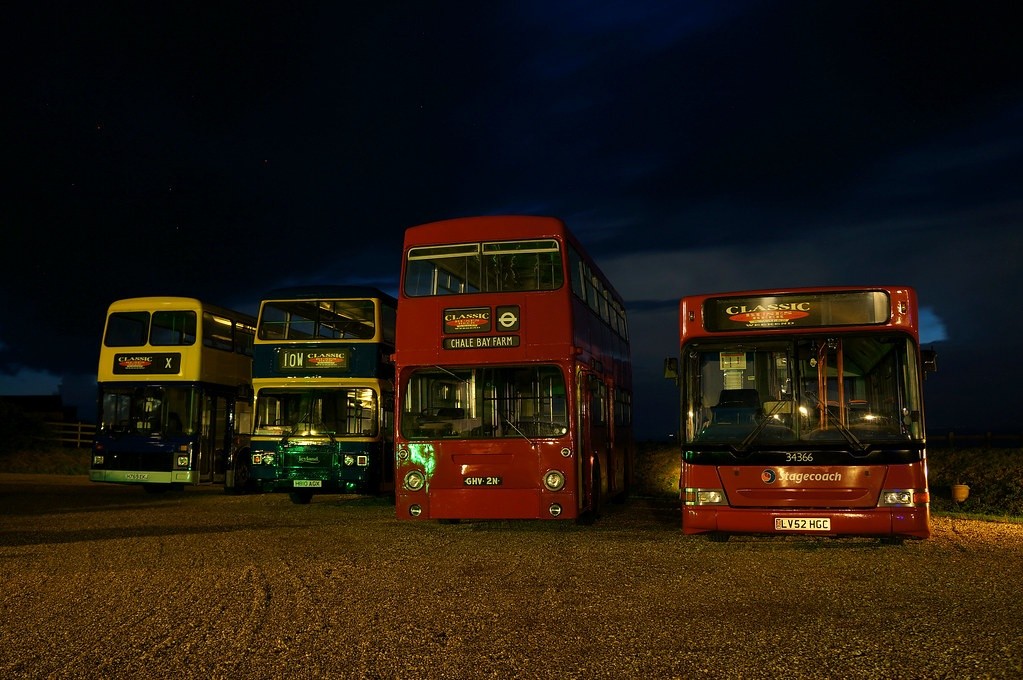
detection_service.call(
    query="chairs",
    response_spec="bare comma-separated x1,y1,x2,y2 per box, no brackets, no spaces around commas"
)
817,399,868,411
436,408,464,419
709,389,765,427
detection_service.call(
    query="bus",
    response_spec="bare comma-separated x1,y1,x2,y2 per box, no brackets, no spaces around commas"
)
88,296,259,485
247,293,400,504
391,213,634,525
662,285,939,546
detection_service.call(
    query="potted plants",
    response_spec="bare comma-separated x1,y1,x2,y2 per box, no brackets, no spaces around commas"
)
937,450,975,503
